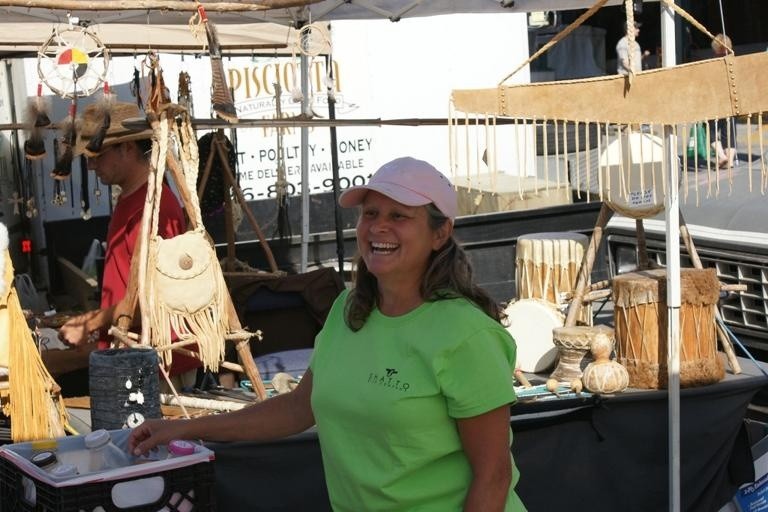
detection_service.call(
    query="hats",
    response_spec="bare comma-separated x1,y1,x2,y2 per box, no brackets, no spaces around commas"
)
61,102,153,156
624,21,642,29
338,157,457,225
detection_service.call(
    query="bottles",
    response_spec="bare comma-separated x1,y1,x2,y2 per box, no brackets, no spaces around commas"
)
85,428,131,473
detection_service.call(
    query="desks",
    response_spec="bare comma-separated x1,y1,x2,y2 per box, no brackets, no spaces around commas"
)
64,351,768,512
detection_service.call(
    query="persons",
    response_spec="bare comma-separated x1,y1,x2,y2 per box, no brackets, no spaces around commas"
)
615,20,651,75
700,33,737,170
56,101,204,396
125,156,533,512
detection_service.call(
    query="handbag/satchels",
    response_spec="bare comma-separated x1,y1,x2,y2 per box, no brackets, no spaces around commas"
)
145,232,217,314
687,127,707,161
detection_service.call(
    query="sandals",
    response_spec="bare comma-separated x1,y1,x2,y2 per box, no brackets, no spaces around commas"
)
712,158,734,170
